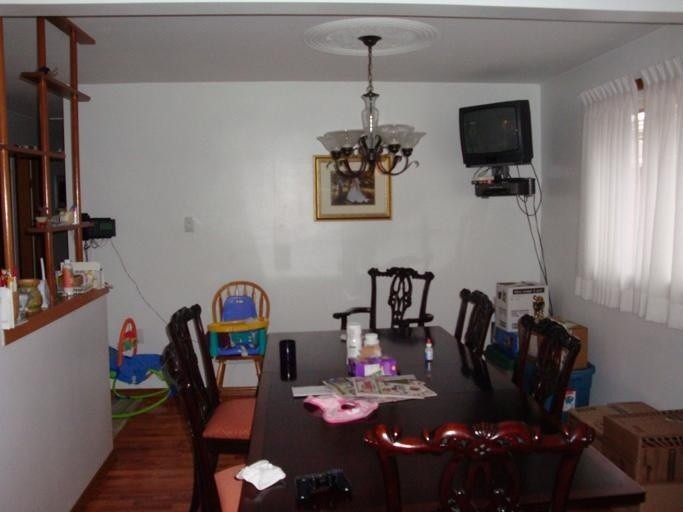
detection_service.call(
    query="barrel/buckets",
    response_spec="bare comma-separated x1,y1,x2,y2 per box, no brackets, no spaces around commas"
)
540,362,596,413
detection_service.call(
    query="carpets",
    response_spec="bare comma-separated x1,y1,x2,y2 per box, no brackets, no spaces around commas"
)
111,394,141,441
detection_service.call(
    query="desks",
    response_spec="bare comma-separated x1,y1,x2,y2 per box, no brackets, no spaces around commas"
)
237,324,647,512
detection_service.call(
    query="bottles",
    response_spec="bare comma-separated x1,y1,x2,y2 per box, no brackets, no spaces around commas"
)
62,258,75,296
345,322,362,365
424,338,433,360
362,333,382,356
424,361,432,378
0,269,17,293
17,278,43,312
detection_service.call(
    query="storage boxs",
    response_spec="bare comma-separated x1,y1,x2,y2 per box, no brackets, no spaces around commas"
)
492,322,520,357
524,361,596,422
566,400,683,512
528,315,588,371
495,281,550,332
484,344,514,371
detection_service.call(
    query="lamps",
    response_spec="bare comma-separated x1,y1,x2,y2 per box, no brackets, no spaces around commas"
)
317,35,426,178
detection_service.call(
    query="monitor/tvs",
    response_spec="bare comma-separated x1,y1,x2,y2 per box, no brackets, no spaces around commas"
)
459,100,533,168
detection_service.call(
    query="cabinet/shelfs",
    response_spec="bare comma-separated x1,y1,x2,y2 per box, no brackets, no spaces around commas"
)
1,15,112,346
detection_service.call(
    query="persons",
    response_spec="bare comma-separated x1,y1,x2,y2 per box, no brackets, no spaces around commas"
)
337,177,348,202
344,178,368,203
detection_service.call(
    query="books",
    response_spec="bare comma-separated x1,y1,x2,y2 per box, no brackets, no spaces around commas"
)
321,371,438,405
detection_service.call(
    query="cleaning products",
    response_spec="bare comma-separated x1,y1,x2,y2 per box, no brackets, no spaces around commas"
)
62,259,73,295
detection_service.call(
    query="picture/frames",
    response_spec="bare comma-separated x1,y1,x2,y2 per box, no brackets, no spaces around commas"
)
312,155,392,221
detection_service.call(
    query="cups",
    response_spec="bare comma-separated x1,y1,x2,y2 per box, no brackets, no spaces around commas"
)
37,279,50,310
280,361,297,381
279,338,296,362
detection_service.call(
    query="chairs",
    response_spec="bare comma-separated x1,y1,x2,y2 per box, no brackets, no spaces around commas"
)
332,265,435,329
511,314,581,420
454,288,495,358
361,418,596,512
206,281,270,387
161,302,256,512
